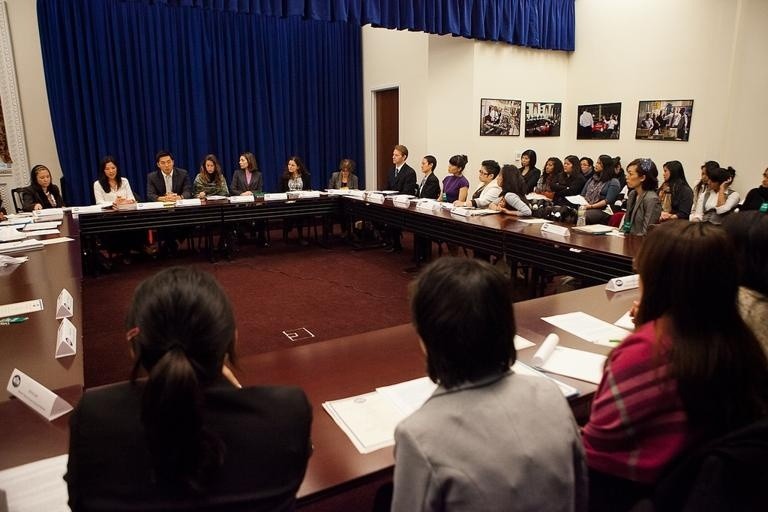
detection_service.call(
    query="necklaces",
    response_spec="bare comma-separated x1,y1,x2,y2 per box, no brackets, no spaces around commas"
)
110,183,117,191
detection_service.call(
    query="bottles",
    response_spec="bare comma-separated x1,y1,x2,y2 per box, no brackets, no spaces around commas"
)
576,202,586,227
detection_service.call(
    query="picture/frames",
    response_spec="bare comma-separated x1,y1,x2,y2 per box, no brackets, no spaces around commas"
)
479,98,521,136
636,100,693,141
525,101,562,137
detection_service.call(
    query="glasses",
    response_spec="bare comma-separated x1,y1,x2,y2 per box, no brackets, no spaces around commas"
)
480,170,488,175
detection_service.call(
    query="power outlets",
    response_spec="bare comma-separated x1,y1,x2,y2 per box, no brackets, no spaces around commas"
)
515,152,521,161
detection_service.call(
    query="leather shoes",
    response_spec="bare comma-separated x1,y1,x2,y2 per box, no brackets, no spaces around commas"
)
123,252,131,265
328,230,348,239
248,237,272,248
209,249,232,263
384,244,400,252
284,224,308,245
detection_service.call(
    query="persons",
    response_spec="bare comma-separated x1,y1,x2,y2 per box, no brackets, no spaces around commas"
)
93,155,135,260
0,195,8,221
328,159,358,235
147,151,192,257
63,265,313,510
229,153,264,244
192,154,229,249
640,108,688,139
721,210,767,359
579,107,618,139
22,165,64,212
280,155,311,242
390,256,591,511
386,145,768,294
580,221,767,510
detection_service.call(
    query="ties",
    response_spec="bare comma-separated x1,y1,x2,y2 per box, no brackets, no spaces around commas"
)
394,169,399,177
